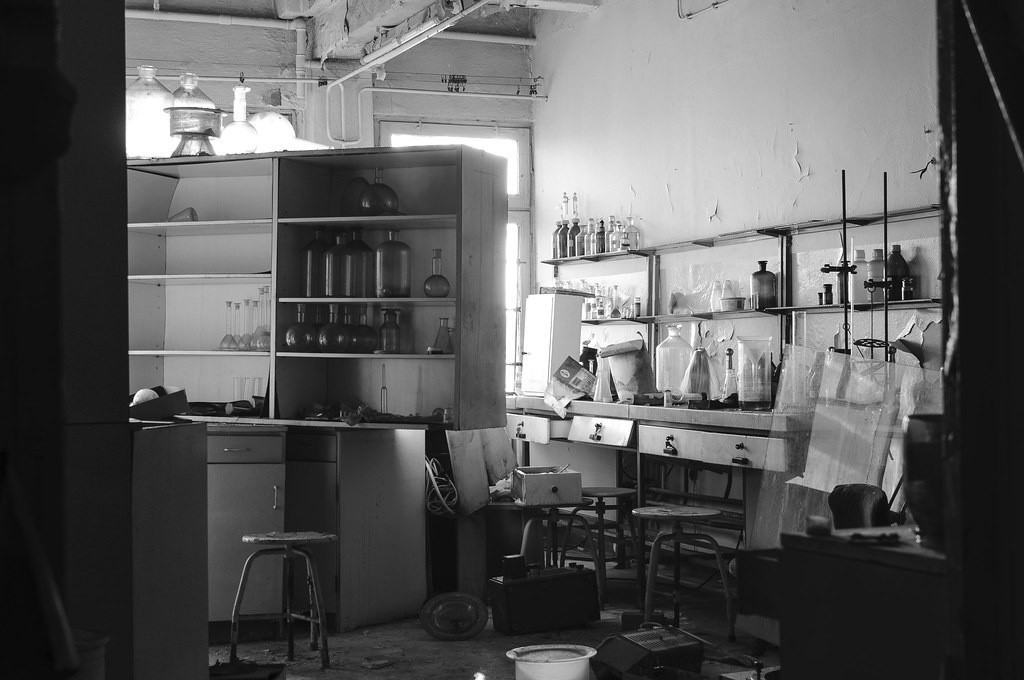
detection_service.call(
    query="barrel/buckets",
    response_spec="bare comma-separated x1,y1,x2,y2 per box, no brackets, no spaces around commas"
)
505,644,597,680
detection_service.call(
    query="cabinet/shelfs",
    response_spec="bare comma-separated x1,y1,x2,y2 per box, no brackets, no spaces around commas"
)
209,436,287,621
534,203,950,405
128,145,509,431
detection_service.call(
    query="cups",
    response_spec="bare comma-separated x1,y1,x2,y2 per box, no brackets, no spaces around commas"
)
803,515,832,538
443,408,453,423
735,335,772,409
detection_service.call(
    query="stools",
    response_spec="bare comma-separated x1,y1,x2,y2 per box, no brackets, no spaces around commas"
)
230,530,337,667
560,488,650,610
632,505,738,638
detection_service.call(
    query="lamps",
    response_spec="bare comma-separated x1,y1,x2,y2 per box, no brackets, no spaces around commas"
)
220,72,258,152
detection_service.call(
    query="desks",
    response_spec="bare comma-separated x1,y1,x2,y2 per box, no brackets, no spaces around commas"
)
777,525,962,680
485,499,606,618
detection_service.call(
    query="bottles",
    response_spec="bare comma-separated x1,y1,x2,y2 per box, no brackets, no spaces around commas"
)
286,303,455,354
126,64,257,158
231,375,261,407
219,286,271,352
300,227,451,298
656,323,737,409
818,244,915,305
166,207,199,222
554,278,641,318
550,191,641,260
901,411,945,548
420,593,489,639
710,260,778,311
579,346,612,402
